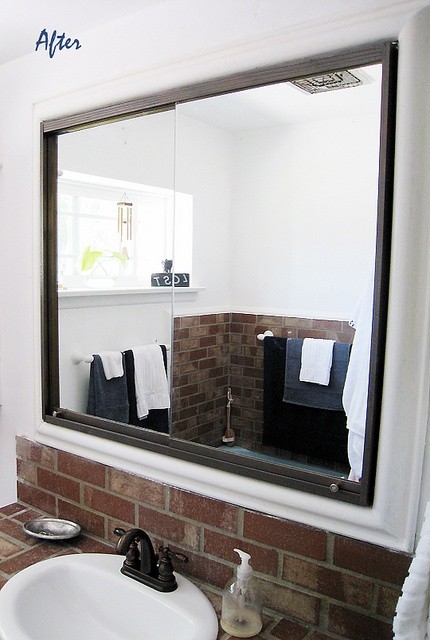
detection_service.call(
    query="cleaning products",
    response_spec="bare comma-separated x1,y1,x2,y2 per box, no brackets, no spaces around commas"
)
220,548,263,637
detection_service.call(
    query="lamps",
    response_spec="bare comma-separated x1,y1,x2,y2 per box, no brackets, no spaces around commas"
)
286,69,374,97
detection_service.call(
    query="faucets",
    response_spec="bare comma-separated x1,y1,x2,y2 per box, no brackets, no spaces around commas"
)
116,528,156,575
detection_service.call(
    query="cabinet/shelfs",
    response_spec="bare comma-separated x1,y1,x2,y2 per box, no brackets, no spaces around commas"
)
58,166,208,296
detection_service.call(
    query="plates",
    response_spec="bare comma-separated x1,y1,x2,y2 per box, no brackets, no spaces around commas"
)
21,515,83,546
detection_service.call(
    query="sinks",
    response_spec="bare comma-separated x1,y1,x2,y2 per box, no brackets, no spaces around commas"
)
12,564,197,640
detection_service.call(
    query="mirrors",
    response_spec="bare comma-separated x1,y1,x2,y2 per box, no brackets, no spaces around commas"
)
32,2,429,555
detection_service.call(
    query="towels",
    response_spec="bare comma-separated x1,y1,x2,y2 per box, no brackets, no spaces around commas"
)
91,351,125,381
87,353,129,425
124,346,170,436
263,337,349,467
283,335,352,418
127,345,172,421
299,336,336,386
